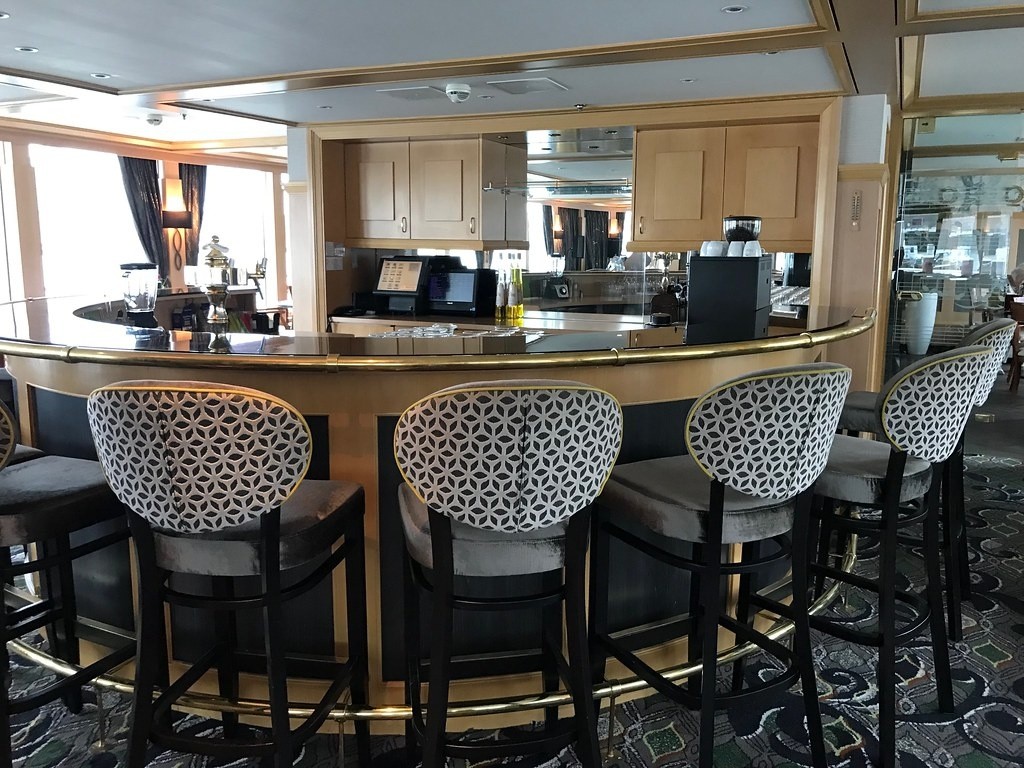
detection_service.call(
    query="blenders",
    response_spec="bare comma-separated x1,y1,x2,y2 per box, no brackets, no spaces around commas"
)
120,263,159,328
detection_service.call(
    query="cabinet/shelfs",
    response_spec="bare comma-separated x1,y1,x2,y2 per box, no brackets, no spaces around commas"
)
344,138,508,251
626,120,820,254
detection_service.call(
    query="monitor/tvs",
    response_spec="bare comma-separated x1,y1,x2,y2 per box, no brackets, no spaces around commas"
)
372,258,430,296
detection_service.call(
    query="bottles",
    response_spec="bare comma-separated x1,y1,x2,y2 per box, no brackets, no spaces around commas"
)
518,268,522,318
508,268,517,319
495,272,505,318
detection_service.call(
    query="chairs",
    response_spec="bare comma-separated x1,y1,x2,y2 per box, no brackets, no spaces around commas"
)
1007,301,1024,393
87,380,370,768
732,345,996,768
0,399,174,768
588,362,853,768
393,377,623,768
815,319,1018,642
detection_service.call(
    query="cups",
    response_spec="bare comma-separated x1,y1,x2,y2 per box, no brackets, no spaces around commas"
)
700,241,762,257
